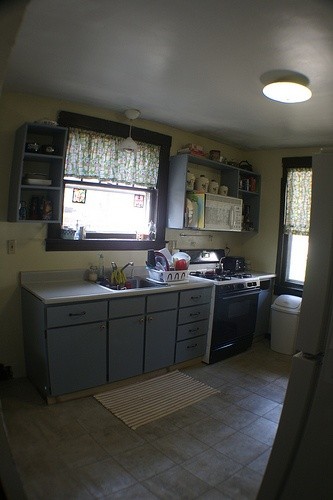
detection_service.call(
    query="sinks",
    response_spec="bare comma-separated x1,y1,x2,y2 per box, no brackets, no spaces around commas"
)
96,277,167,291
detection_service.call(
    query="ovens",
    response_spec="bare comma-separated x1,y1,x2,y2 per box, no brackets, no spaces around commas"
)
202,278,261,365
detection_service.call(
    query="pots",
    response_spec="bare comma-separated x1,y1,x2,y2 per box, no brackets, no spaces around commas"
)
225,256,246,273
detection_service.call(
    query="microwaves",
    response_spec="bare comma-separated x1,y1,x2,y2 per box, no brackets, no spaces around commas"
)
187,192,243,232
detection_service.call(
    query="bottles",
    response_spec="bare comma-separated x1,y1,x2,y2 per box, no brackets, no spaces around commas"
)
27,126,40,152
220,184,228,196
219,155,232,166
244,206,254,224
195,174,209,192
18,194,53,220
79,227,84,240
209,179,219,194
89,266,97,281
186,170,195,191
98,254,105,280
240,174,257,192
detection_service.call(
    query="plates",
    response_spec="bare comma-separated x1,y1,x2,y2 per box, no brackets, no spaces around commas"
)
154,251,170,271
24,178,52,185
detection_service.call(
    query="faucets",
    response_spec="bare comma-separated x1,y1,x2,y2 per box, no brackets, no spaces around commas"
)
111,261,134,281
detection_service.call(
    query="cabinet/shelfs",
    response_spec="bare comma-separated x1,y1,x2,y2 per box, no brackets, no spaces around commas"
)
167,154,260,234
8,122,68,224
21,288,213,398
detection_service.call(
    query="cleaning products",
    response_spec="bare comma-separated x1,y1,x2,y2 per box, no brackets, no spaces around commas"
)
88,253,105,283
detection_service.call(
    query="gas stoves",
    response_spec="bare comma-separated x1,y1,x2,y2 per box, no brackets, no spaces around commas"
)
181,247,255,283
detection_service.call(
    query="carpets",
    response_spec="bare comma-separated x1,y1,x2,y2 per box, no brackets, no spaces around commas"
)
93,369,220,430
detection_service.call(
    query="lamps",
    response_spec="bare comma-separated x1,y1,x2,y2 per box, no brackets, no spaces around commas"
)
263,80,312,103
121,110,140,152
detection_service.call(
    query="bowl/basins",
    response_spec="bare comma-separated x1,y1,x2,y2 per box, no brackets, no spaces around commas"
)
62,229,76,239
173,252,191,267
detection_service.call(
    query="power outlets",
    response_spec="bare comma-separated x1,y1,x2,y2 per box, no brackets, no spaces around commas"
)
225,242,230,250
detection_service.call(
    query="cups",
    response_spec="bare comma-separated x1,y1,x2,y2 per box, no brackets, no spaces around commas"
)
216,263,224,280
175,259,187,270
160,248,173,266
147,249,155,267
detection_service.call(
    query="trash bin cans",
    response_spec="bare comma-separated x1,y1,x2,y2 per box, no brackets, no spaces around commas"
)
269,293,302,356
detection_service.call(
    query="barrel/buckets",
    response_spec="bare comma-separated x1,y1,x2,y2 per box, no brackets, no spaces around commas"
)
209,149,220,162
